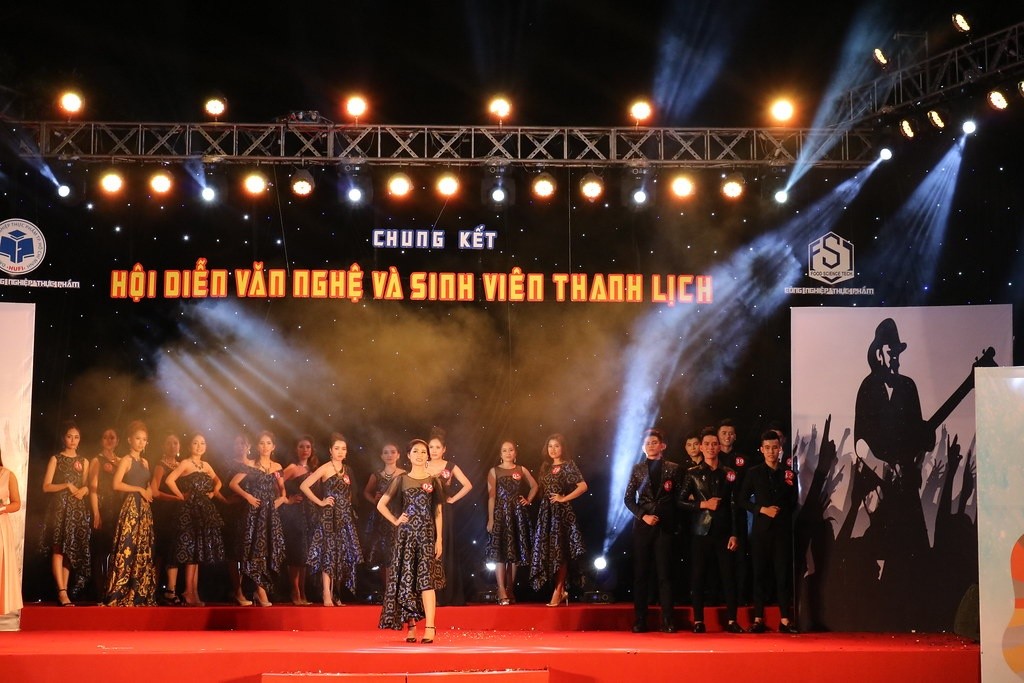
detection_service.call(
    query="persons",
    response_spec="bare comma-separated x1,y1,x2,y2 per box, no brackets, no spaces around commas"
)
681,430,703,471
737,430,799,633
479,438,539,606
300,431,358,606
376,438,446,643
214,430,255,593
164,427,222,607
229,431,285,606
676,427,745,633
0,446,24,616
530,432,588,606
426,424,473,606
94,417,158,606
766,420,791,469
151,427,182,606
35,419,89,606
282,434,319,605
89,425,122,606
717,418,751,475
624,426,685,633
363,439,408,602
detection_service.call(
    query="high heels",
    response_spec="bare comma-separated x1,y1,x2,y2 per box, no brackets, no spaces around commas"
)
160,589,182,606
231,595,252,606
290,593,304,606
499,597,510,606
333,594,346,607
421,626,436,644
253,592,273,606
299,593,312,605
406,625,417,643
58,589,74,606
545,592,569,607
181,591,204,607
322,592,335,607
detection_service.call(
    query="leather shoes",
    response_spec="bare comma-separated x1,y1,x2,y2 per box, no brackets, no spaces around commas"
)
633,623,644,632
749,621,765,633
779,620,801,634
726,621,745,633
695,622,708,631
663,623,675,632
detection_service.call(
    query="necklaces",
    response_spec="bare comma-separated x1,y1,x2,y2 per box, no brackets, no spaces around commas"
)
258,459,271,473
164,457,177,469
384,467,397,478
102,452,117,463
298,463,308,468
191,458,204,470
331,461,345,476
